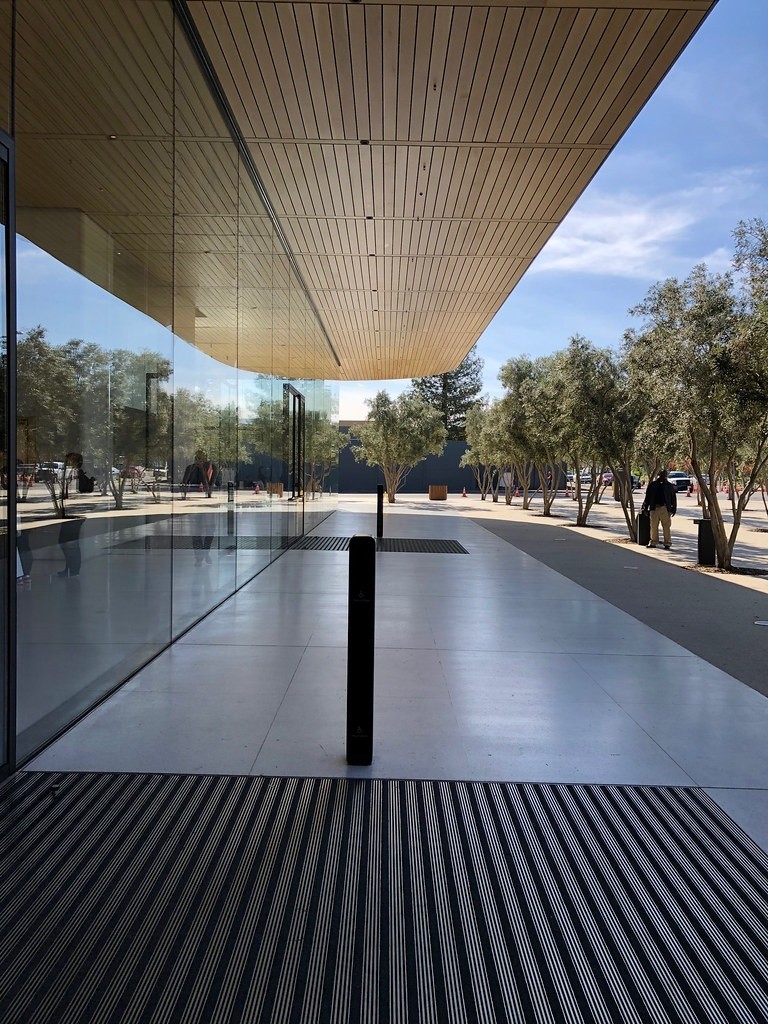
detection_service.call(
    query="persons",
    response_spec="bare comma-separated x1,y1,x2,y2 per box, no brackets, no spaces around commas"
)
179,450,222,568
641,469,676,549
546,471,552,490
0,452,85,585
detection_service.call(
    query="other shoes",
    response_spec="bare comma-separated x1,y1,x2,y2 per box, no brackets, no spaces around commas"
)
205,554,212,564
194,559,203,568
57,567,78,578
665,545,670,549
15,575,34,585
646,544,657,548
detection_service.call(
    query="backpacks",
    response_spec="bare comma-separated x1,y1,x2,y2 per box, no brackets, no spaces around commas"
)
75,468,96,511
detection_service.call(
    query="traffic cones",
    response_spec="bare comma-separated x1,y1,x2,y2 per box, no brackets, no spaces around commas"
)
252,485,260,494
461,486,467,497
564,488,570,497
514,487,521,497
686,487,691,497
198,482,204,492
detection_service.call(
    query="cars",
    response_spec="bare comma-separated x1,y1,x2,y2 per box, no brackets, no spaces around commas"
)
572,471,592,484
596,473,614,486
153,468,165,477
122,466,146,478
631,475,642,489
566,470,573,481
102,467,122,481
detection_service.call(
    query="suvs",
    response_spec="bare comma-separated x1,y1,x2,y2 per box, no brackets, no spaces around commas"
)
666,471,711,493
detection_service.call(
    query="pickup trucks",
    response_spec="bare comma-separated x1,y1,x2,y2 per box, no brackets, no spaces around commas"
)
34,460,75,483
